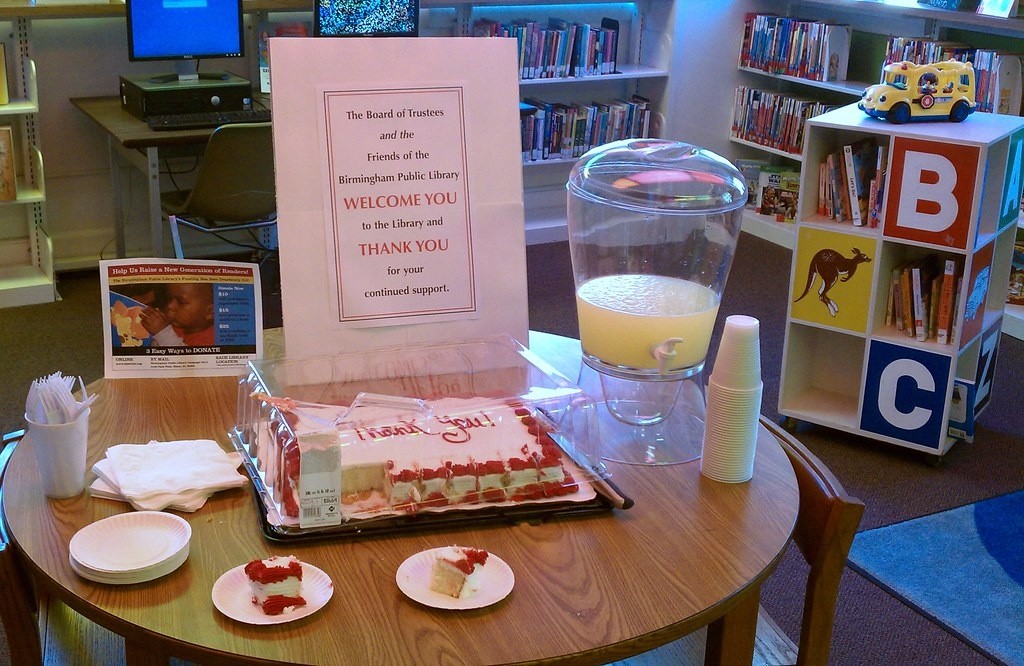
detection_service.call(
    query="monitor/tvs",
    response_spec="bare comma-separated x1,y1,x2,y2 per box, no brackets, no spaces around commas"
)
126,0,245,84
313,0,420,37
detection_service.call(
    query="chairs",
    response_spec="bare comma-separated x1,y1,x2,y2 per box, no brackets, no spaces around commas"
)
603,415,864,666
0,429,199,666
160,122,278,258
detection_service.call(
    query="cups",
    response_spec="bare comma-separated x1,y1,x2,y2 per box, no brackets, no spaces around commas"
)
700,315,764,485
24,401,90,500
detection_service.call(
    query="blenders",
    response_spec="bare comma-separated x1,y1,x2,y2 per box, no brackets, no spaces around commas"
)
561,137,750,466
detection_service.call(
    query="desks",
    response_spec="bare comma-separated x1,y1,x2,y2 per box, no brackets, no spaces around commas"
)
3,329,802,666
69,86,272,258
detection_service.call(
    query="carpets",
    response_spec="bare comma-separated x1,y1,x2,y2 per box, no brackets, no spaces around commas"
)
846,489,1024,666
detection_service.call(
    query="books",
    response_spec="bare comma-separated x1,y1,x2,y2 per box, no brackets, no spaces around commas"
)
885,37,1024,116
474,17,617,79
818,137,889,228
731,85,844,158
520,95,651,163
741,13,852,82
886,259,963,346
734,158,801,225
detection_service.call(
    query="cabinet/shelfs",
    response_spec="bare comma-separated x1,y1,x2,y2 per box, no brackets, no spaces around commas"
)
0,20,54,310
420,0,671,245
776,102,1024,466
726,0,1024,342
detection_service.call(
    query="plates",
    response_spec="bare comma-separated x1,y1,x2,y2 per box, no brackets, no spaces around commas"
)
211,561,334,626
396,547,515,610
68,510,193,585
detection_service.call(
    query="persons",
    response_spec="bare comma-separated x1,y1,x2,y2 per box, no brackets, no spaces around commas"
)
139,283,215,346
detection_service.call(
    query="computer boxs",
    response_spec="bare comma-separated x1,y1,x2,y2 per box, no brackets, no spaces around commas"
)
119,70,252,123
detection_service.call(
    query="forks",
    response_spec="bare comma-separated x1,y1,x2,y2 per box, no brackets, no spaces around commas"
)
25,371,99,424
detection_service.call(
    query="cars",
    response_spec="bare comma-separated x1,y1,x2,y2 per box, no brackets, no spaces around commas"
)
857,55,977,126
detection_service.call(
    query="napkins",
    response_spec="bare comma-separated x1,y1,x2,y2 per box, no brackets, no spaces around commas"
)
88,438,249,513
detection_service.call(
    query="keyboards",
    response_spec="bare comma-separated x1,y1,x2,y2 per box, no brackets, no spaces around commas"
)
148,108,273,132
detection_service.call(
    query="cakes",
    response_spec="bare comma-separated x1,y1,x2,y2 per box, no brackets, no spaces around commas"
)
244,555,306,616
431,546,488,598
254,391,578,510
